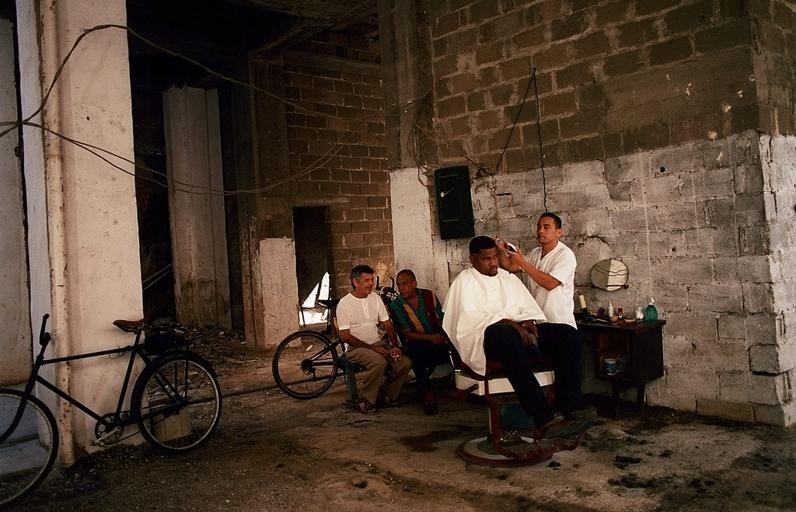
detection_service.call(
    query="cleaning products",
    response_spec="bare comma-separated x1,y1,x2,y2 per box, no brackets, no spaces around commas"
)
644,297,658,321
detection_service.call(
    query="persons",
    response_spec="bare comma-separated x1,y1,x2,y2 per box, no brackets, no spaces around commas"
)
336,264,413,415
442,236,596,432
388,269,460,416
496,212,582,331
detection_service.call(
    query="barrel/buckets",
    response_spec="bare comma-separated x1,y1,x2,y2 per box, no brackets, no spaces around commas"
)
149,398,191,442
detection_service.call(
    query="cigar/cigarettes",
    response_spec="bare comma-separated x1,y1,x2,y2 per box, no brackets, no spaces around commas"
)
393,355,399,358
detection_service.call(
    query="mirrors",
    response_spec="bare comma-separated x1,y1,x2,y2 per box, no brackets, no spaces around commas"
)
588,258,629,290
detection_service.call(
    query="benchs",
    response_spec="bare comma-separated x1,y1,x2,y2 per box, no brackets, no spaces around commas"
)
334,347,450,411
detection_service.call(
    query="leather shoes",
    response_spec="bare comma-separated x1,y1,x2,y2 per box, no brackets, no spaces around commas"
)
377,384,401,406
355,397,376,414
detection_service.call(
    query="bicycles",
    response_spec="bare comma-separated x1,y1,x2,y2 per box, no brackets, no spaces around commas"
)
272,276,401,401
0,313,223,509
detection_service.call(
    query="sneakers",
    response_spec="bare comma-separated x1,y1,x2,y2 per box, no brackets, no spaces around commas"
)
421,388,438,415
534,408,565,430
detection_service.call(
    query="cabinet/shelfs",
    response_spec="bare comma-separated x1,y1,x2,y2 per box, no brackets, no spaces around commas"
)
574,314,667,421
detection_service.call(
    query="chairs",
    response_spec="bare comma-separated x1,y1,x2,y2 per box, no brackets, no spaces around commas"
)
445,332,606,467
